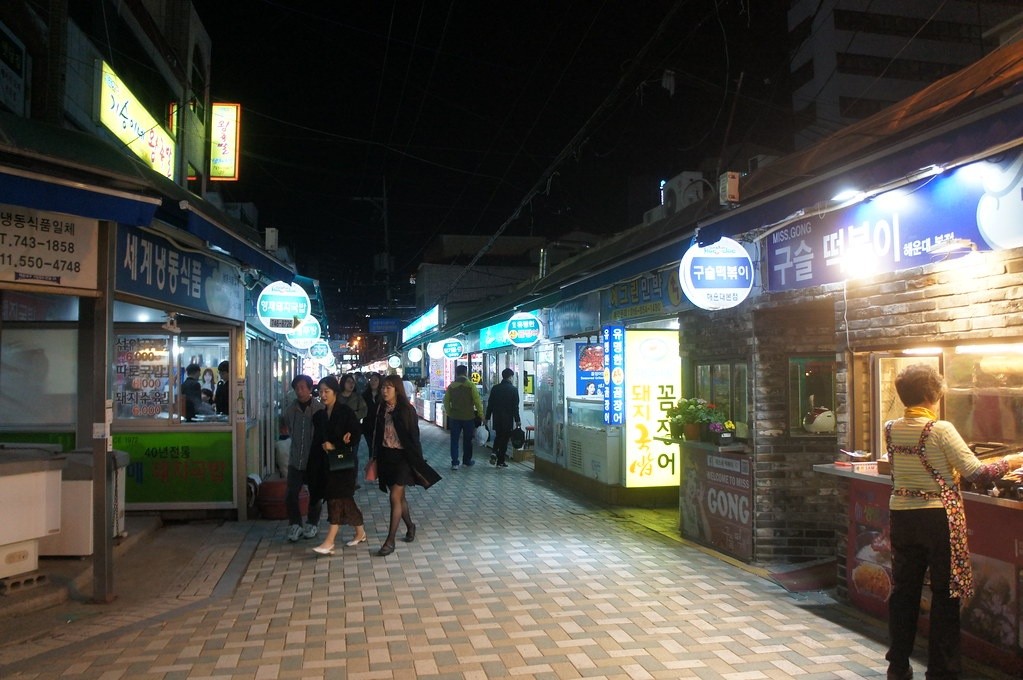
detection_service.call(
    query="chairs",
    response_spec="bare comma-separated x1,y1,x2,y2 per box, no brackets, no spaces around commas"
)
524,426,535,449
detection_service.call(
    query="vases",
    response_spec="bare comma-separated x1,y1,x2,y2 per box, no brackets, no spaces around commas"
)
669,423,733,447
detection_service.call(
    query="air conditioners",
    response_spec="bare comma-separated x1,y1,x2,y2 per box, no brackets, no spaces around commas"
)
748,154,779,173
643,170,704,227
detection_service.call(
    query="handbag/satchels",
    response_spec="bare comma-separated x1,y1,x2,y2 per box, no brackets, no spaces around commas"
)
328,449,356,472
365,460,378,481
511,425,525,449
474,423,490,447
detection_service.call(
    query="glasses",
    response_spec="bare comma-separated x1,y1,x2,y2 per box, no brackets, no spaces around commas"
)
217,370,226,373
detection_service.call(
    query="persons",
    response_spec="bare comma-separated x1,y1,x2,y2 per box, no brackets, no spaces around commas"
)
485,368,522,469
343,375,441,556
283,375,325,542
585,383,603,395
885,367,1023,680
313,377,366,555
200,369,217,401
181,360,229,423
443,366,484,470
332,372,429,453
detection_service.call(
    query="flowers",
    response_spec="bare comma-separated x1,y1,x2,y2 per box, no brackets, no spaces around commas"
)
666,397,735,433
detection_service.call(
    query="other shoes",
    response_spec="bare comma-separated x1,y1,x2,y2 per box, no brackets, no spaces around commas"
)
312,543,336,555
497,463,508,467
452,462,459,469
886,665,913,680
347,532,366,546
490,454,496,465
378,542,395,555
462,460,475,467
405,523,415,542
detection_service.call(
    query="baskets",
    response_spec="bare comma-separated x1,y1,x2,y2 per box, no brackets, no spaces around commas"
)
877,458,890,476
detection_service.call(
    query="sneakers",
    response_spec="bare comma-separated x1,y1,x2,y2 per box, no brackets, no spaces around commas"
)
303,522,318,538
287,523,303,542
